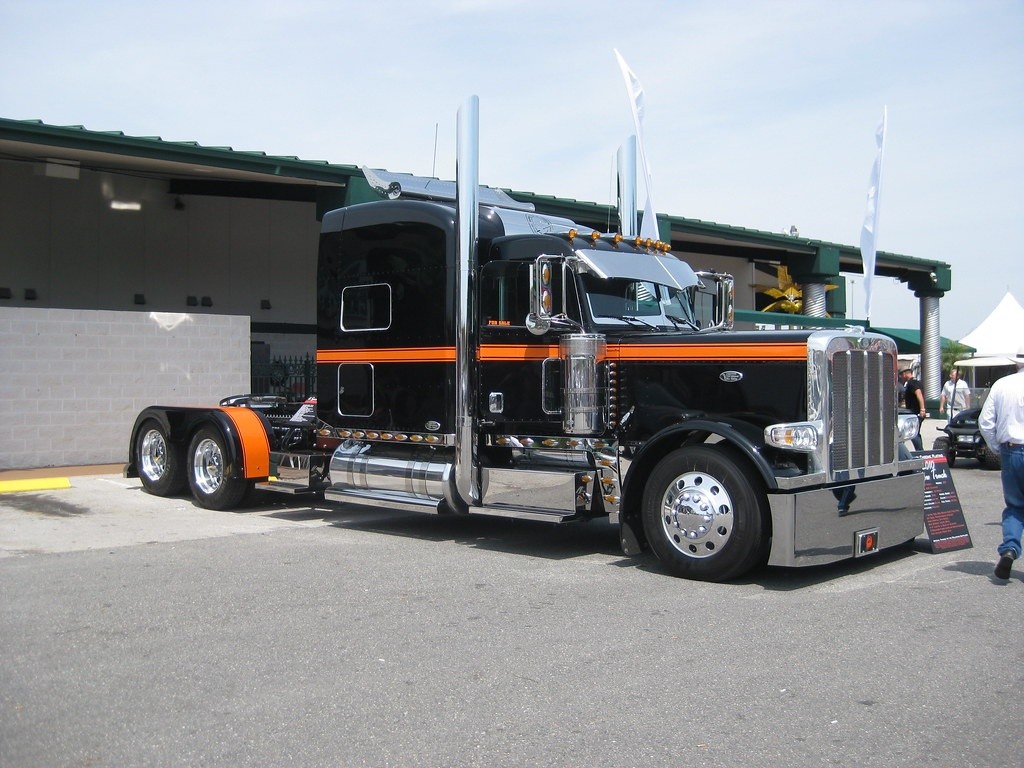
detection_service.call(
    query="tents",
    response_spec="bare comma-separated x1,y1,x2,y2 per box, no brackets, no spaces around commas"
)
958,292,1024,380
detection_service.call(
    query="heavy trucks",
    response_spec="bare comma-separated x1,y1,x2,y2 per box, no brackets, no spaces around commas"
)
121,199,925,587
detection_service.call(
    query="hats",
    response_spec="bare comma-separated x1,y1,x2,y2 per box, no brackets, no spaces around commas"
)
1009,354,1024,364
899,366,910,374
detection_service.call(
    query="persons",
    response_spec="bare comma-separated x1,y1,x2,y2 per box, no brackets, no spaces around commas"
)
899,443,914,476
898,366,926,451
939,368,971,425
978,342,1024,580
898,382,905,408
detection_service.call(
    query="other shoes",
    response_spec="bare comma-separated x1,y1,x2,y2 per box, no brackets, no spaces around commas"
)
994,550,1015,579
838,508,847,516
848,494,856,505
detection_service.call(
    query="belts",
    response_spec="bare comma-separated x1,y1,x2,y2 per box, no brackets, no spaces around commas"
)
1001,443,1024,448
